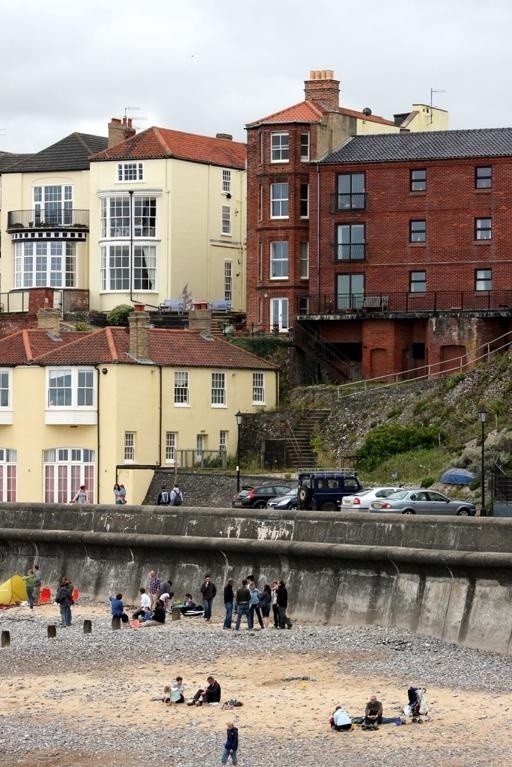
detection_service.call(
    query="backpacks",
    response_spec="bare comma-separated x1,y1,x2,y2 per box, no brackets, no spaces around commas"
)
161,491,169,503
173,490,182,506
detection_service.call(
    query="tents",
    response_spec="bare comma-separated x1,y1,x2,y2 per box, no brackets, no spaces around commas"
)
0,574,28,607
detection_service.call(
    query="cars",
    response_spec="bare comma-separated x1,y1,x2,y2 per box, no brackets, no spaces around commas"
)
266,486,298,509
340,487,406,511
231,484,293,509
368,489,476,518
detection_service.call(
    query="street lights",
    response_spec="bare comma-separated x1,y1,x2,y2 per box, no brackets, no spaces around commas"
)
477,408,488,517
235,409,244,492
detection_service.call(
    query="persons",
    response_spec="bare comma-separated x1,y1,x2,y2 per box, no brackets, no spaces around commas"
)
165,675,221,707
221,721,240,766
22,565,75,627
329,706,352,732
361,694,383,731
111,480,184,506
110,570,292,633
71,485,89,505
404,687,428,723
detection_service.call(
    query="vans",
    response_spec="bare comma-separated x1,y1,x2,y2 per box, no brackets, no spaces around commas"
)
296,471,363,511
